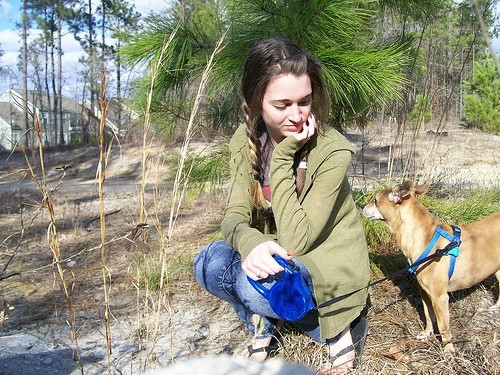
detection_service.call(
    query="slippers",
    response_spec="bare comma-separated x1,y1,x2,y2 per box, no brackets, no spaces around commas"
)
317,316,367,375
245,330,282,363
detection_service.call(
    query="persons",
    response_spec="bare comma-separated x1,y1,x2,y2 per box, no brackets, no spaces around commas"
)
193,37,371,375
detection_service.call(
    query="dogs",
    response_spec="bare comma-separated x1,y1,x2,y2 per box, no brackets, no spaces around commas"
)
363,178,500,352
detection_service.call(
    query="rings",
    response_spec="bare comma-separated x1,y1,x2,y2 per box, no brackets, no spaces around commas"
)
256,269,263,278
